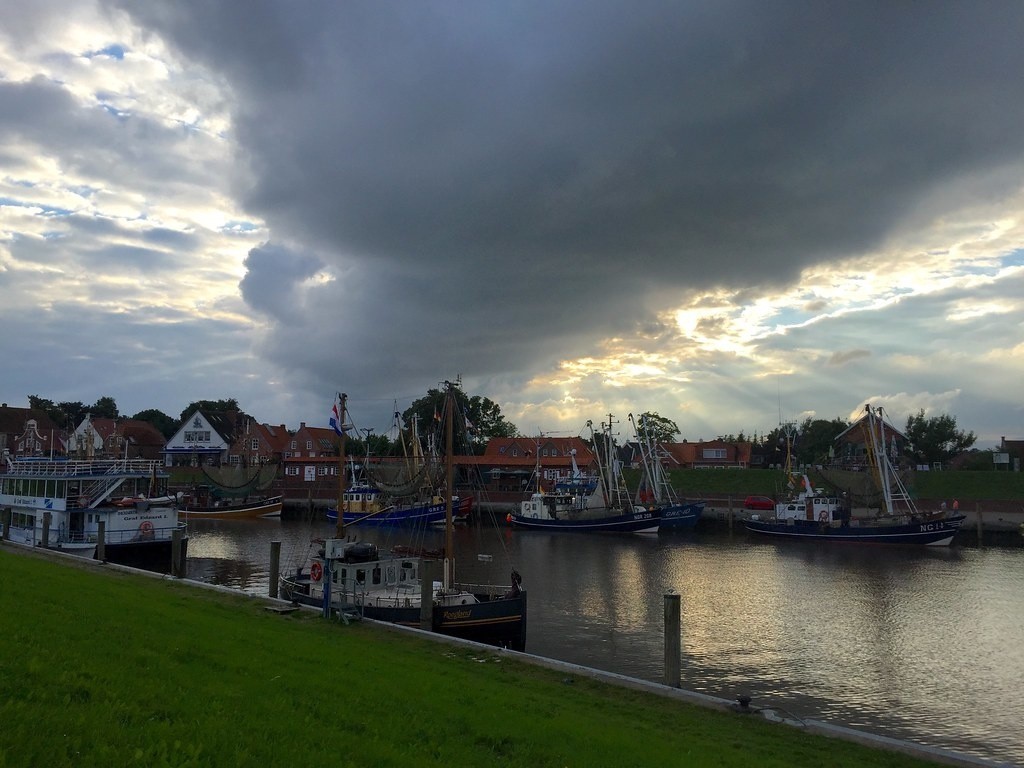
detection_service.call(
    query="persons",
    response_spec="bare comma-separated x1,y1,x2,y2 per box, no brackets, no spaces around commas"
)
522,477,527,491
952,499,959,517
942,499,946,513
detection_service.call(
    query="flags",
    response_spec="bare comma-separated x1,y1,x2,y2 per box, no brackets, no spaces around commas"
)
434,404,441,422
463,403,481,434
329,402,343,439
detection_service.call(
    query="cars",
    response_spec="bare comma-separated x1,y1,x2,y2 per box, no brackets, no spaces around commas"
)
744,495,774,510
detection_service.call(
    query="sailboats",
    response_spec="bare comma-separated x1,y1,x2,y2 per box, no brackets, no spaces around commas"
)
632,413,707,530
742,404,967,548
393,410,475,522
0,412,188,575
275,373,527,654
325,399,460,528
177,483,284,521
506,412,668,533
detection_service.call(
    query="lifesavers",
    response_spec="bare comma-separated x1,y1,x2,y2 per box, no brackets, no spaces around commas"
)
310,563,322,581
818,510,830,520
77,494,91,510
140,521,153,535
